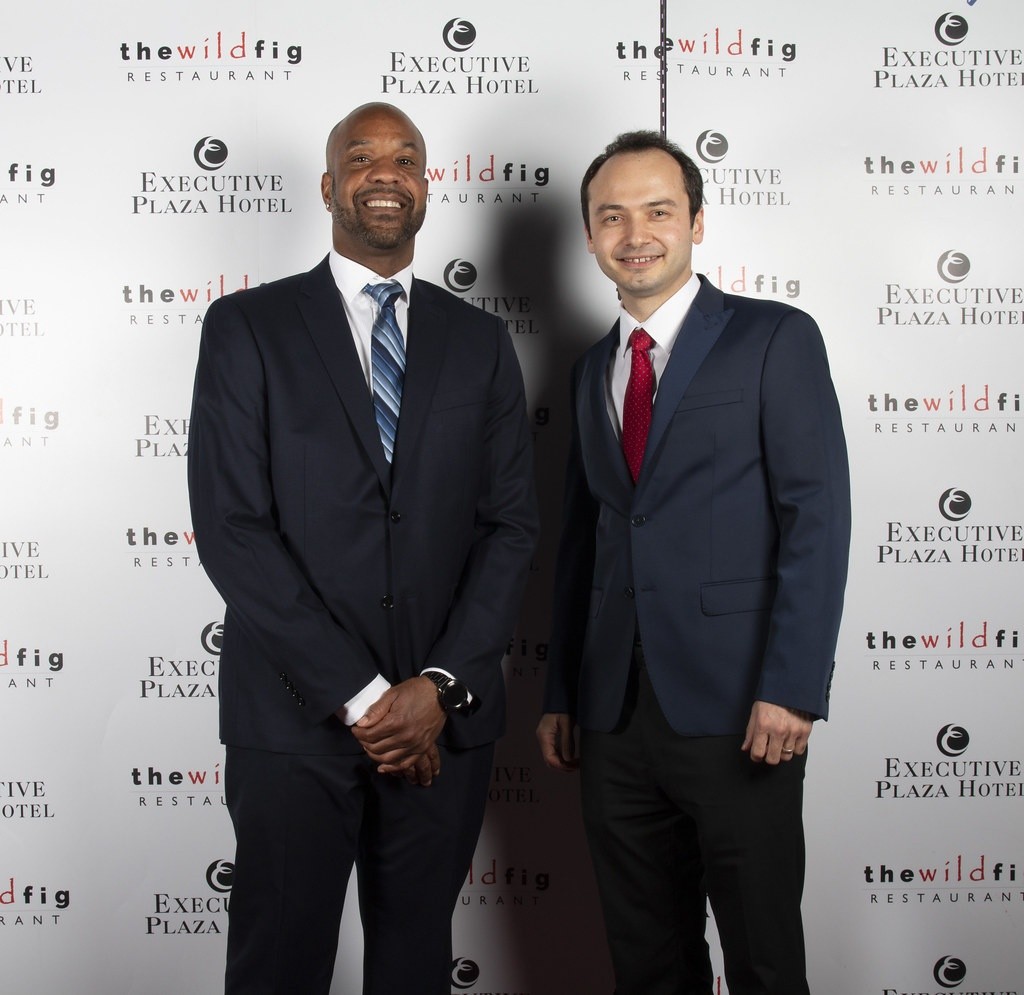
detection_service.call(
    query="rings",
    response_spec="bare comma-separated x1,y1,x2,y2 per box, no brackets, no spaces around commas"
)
782,746,795,753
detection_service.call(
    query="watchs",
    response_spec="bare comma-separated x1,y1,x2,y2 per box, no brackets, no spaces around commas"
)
424,670,468,721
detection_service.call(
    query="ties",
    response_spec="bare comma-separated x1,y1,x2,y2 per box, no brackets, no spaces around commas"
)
362,282,407,474
622,329,653,482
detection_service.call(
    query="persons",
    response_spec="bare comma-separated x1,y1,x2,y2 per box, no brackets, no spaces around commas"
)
532,129,852,992
186,103,527,994
